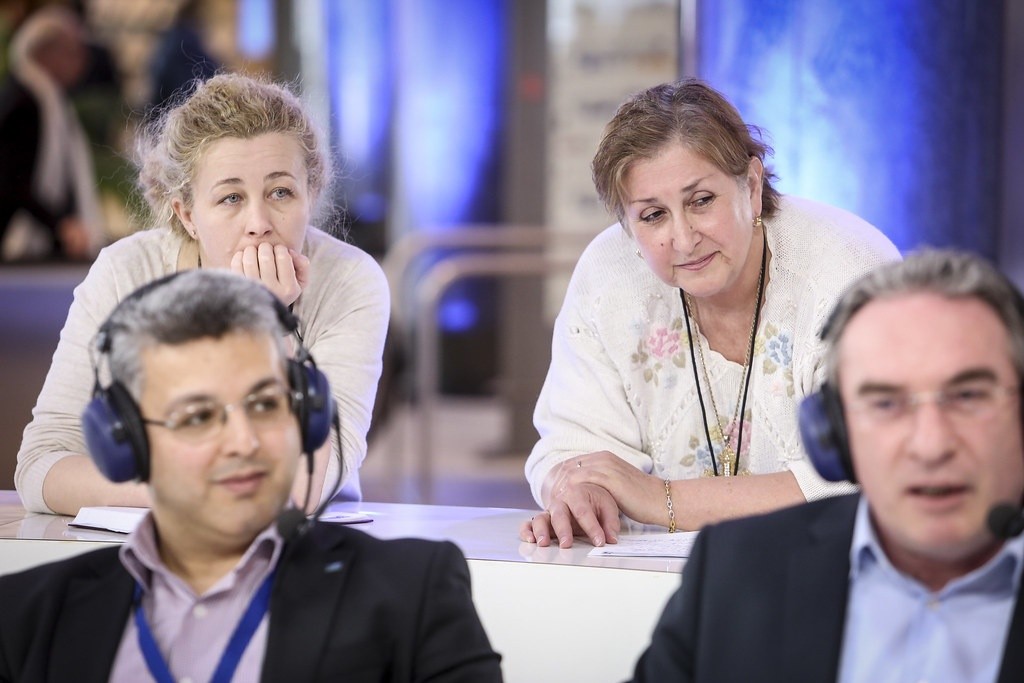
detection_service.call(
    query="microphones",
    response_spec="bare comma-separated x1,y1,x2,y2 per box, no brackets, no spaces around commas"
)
273,422,341,544
986,489,1024,540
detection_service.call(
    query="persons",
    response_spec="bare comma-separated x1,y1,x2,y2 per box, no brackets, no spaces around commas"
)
621,247,1024,683
13,74,391,516
0,269,506,683
516,82,904,549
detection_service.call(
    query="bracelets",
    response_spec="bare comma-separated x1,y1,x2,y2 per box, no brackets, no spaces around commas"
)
664,478,676,533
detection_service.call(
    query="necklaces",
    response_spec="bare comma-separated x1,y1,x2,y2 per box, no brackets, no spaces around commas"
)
683,232,767,476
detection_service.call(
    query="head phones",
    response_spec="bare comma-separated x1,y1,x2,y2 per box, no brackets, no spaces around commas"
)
795,282,1023,484
82,271,341,484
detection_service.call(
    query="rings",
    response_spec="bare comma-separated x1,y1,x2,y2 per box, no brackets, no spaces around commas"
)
577,461,582,466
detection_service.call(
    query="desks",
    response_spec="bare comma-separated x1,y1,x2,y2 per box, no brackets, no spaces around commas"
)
0,265,90,489
0,485,686,683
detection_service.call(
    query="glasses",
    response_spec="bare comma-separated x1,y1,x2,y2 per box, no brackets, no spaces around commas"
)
837,379,1024,424
142,388,305,433
